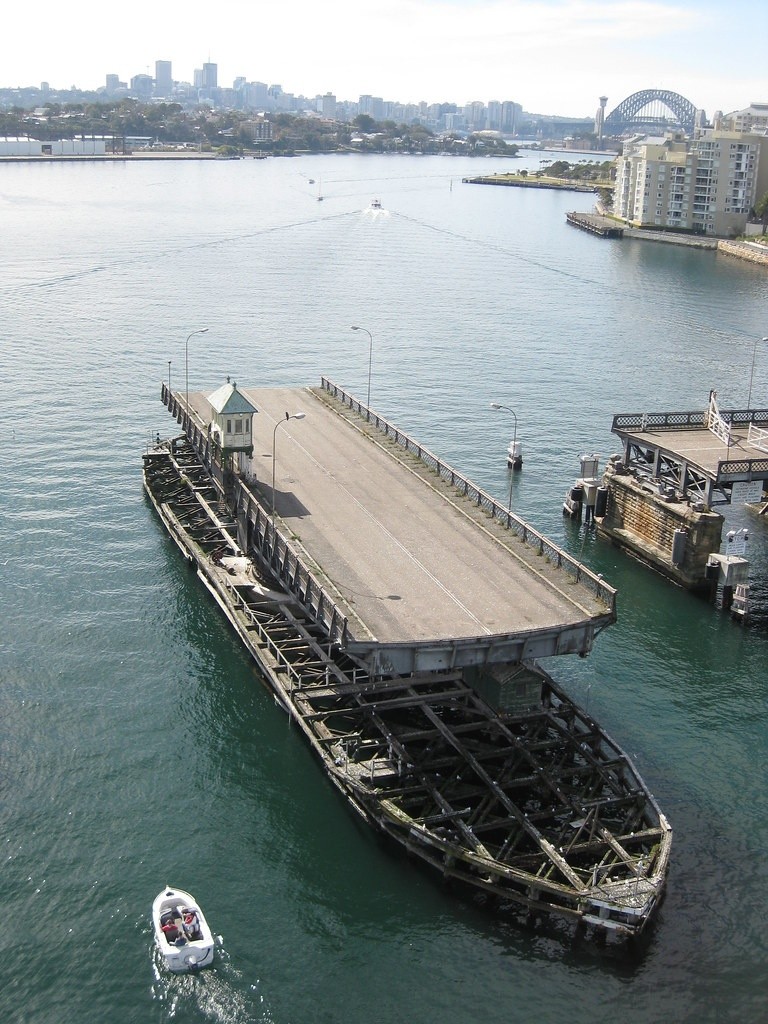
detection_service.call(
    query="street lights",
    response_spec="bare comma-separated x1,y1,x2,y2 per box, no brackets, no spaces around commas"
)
747,337,768,410
185,327,210,415
271,412,307,534
490,403,518,513
350,326,373,408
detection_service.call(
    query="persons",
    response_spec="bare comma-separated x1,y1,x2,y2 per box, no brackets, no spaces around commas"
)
183,912,199,935
160,919,187,946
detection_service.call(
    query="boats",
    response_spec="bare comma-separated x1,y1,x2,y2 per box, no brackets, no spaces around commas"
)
140,322,676,946
150,884,216,973
